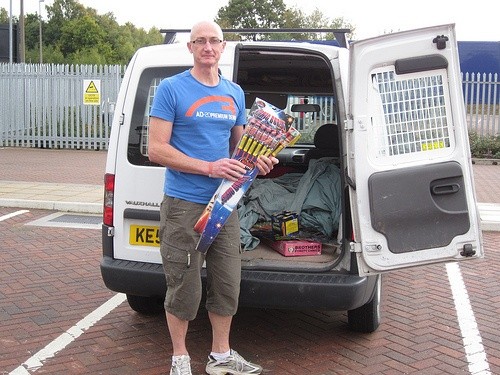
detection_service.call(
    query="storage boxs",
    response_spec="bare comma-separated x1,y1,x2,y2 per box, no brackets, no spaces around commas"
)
271,210,299,237
261,235,322,257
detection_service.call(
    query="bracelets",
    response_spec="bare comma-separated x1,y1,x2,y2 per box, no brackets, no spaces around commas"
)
209,162,213,178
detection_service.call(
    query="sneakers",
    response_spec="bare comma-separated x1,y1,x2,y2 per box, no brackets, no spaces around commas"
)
206,349,263,375
169,355,192,375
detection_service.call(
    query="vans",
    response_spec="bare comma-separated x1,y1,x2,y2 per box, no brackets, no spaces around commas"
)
98,22,486,335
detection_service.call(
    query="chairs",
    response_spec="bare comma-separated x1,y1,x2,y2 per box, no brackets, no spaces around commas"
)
302,124,340,173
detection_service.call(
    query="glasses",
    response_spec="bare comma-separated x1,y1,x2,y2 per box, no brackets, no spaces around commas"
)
190,37,223,44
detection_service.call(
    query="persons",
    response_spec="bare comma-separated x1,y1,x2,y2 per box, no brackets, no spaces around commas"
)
148,21,279,375
